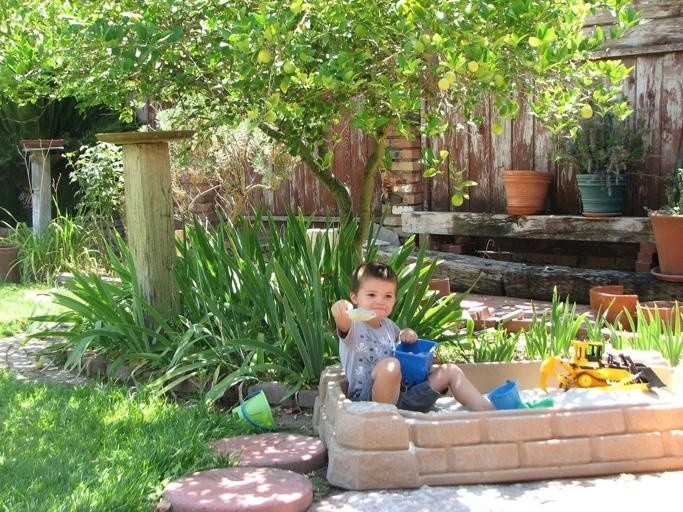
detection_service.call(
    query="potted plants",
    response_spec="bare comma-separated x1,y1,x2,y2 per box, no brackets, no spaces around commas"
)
647,168,683,282
533,59,654,217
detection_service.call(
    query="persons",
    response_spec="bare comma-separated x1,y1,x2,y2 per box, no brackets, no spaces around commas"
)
331,262,496,411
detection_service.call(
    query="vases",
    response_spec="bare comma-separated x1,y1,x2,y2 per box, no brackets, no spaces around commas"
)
589,286,639,330
503,166,550,216
636,298,683,337
